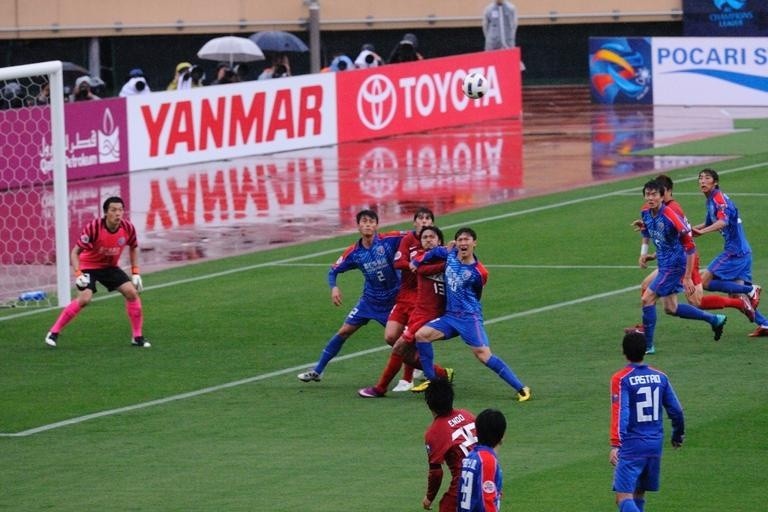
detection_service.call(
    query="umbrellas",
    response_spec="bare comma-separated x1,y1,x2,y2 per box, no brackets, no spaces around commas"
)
248,30,309,52
197,34,264,62
48,59,88,78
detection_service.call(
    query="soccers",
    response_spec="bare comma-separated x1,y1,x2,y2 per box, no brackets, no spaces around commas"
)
463,73,489,99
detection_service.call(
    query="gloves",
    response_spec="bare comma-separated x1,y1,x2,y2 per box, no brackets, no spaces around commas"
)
74,274,90,291
131,274,144,293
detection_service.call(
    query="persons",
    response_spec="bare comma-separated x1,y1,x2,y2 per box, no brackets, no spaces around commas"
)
118,69,148,97
622,175,755,335
44,197,152,349
387,208,436,385
637,179,727,352
458,408,508,512
212,64,235,83
408,227,534,405
167,63,195,89
355,44,382,67
484,0,525,73
424,383,477,511
356,228,455,397
297,211,438,382
683,169,767,337
179,67,202,89
611,329,685,511
259,64,286,79
37,82,49,104
390,33,423,62
328,46,354,70
71,76,95,99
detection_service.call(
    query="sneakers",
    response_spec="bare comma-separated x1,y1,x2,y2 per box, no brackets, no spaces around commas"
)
648,348,651,354
358,387,384,399
409,380,432,394
296,369,324,385
736,291,756,323
709,313,726,343
749,326,768,339
623,325,644,336
444,367,455,383
44,332,58,350
749,283,762,309
131,336,154,349
391,379,417,394
516,385,533,403
